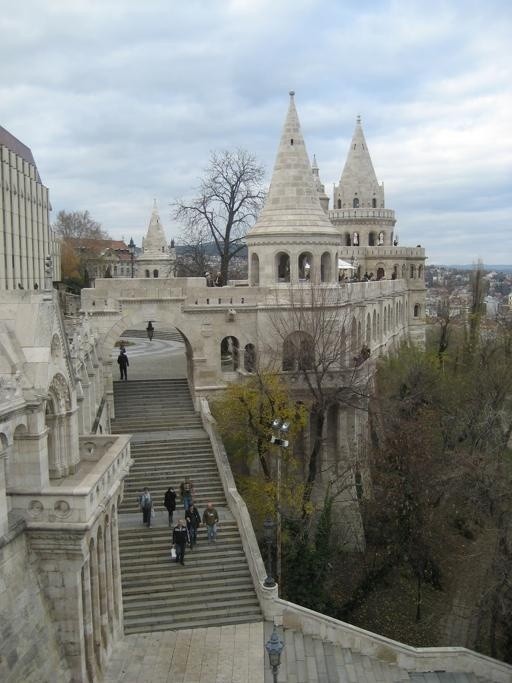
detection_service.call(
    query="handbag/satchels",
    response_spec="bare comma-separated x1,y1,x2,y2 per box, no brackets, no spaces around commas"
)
171,548,177,559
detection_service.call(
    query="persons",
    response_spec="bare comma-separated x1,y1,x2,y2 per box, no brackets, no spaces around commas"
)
337,270,376,281
118,351,129,381
214,271,225,287
184,503,202,549
180,475,195,514
163,488,177,528
203,501,220,545
120,340,126,353
171,519,191,566
137,488,155,529
205,271,214,287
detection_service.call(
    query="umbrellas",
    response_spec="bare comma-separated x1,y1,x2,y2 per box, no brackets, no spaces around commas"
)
305,256,355,270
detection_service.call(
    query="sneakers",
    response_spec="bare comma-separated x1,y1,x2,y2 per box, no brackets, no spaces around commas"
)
181,561,184,564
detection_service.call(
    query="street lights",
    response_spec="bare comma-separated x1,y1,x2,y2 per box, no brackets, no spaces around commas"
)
264,415,292,602
127,236,136,278
